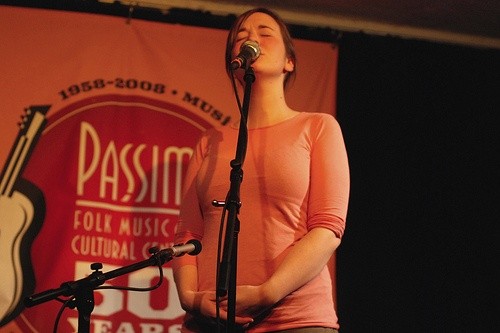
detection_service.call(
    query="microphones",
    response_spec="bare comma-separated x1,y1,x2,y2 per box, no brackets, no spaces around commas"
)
229,41,261,70
161,239,202,263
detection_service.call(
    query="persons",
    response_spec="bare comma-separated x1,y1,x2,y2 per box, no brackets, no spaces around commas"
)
171,8,349,333
